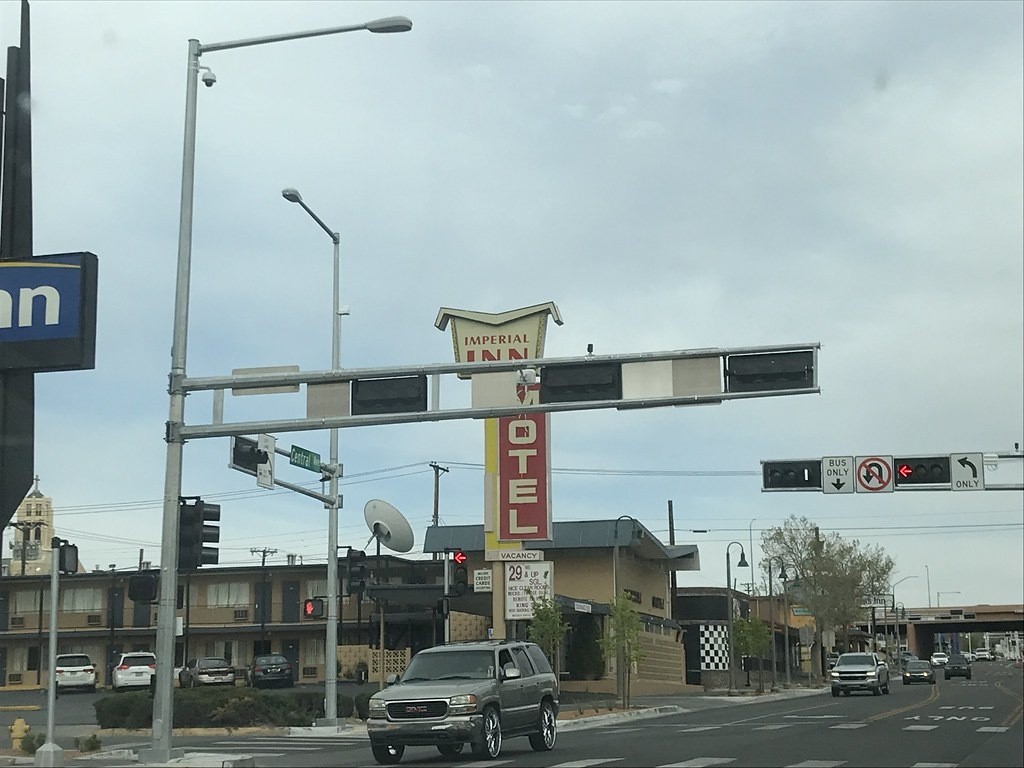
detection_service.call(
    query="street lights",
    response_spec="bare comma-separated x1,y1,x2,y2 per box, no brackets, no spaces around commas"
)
882,565,962,671
138,10,415,765
768,556,789,694
783,566,802,686
615,515,641,700
726,541,750,697
108,563,117,686
279,188,347,728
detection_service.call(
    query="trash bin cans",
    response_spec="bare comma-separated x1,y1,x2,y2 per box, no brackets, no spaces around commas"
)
687,669,700,686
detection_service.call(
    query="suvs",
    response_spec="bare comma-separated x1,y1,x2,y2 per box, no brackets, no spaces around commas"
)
54,653,98,692
106,651,157,693
364,637,560,766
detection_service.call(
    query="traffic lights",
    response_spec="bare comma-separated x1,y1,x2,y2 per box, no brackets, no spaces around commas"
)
302,598,324,618
762,459,823,491
58,544,79,573
177,501,221,574
346,548,368,594
229,434,269,478
452,551,468,596
892,453,952,486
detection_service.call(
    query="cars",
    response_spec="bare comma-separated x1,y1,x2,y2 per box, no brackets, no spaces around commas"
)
827,647,998,698
244,654,294,689
178,656,236,689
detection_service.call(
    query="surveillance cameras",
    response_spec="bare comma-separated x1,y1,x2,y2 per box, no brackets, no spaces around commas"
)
588,344,593,353
1015,443,1018,449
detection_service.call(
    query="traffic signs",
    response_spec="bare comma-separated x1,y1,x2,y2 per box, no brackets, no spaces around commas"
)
256,433,277,490
821,455,855,495
949,452,985,491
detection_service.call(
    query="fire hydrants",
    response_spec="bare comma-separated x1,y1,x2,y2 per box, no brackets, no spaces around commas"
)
8,717,30,751
